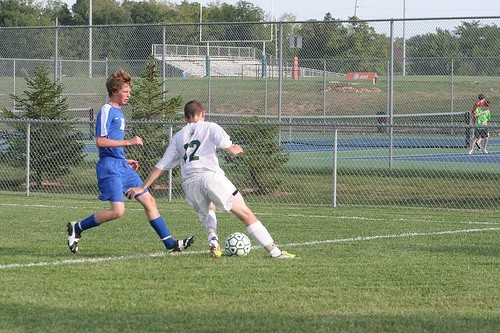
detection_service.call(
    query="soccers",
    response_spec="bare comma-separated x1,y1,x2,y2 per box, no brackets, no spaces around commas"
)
225,232,251,258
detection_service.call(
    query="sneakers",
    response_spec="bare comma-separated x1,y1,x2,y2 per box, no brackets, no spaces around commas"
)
168,236,194,252
209,240,222,258
67,222,81,254
272,251,296,260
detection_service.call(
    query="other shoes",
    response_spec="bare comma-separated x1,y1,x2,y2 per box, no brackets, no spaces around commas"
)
469,149,473,154
475,142,483,151
482,148,488,153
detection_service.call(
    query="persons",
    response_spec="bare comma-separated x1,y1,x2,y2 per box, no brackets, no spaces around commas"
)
66,70,196,255
470,93,486,150
124,100,295,259
468,101,491,155
